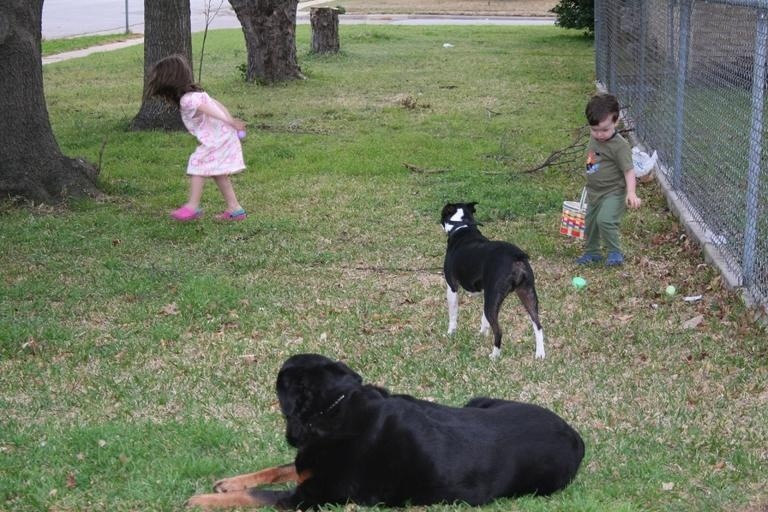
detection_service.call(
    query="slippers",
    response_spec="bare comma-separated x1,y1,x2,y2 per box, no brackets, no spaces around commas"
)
576,254,603,264
605,251,624,266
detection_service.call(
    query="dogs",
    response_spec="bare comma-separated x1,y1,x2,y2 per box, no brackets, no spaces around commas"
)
180,352,587,510
438,199,547,362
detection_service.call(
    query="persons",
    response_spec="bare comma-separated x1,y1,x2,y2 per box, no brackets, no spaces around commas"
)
575,93,642,267
142,55,246,222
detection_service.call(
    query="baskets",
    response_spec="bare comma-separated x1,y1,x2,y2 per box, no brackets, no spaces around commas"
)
560,187,587,239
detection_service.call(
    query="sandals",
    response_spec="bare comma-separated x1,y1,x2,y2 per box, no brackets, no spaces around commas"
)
169,204,203,221
215,208,247,221
